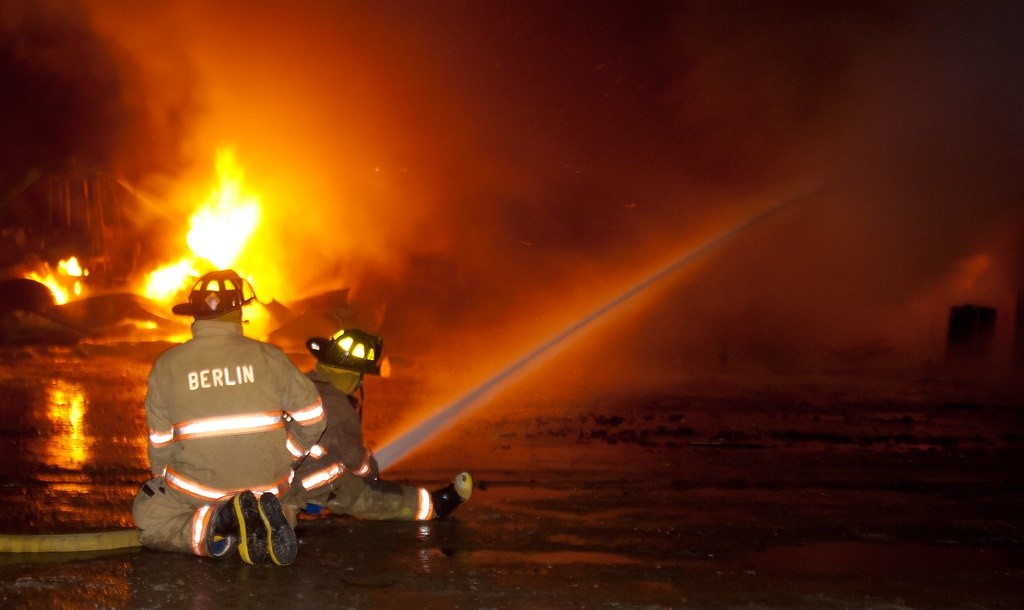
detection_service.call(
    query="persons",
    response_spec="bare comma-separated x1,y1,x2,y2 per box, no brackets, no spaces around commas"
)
131,269,325,568
278,331,472,519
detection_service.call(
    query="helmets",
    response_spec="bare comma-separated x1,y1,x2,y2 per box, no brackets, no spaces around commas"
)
306,329,383,374
172,269,257,320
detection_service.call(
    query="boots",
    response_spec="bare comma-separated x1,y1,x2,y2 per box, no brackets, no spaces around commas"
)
258,492,297,565
430,472,473,518
213,490,267,565
